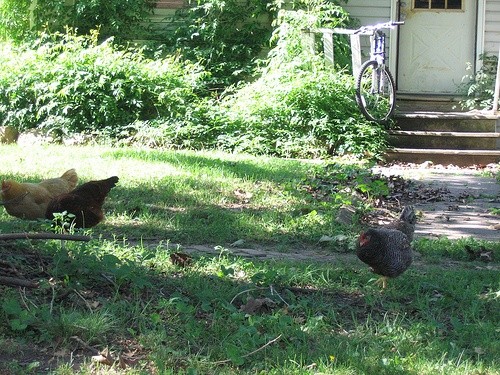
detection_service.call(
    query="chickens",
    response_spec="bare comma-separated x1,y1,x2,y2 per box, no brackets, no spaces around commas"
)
44,176,119,232
0,168,78,220
356,205,417,290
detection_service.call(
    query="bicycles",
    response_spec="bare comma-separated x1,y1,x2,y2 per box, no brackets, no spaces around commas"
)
352,20,406,125
